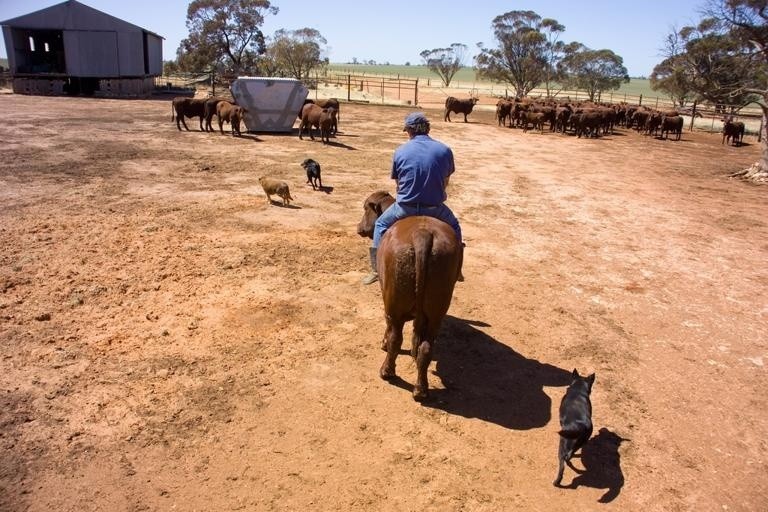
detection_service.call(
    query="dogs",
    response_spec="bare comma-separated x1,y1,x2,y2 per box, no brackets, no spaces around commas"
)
258,176,293,207
300,158,322,190
552,367,595,486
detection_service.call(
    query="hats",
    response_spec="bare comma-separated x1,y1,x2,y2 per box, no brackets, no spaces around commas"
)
404,113,428,132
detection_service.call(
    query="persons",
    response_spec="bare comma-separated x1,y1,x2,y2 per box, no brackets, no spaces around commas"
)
365,110,466,288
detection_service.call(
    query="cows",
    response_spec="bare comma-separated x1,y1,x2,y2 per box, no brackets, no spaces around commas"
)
495,95,683,141
444,96,479,123
356,190,460,401
299,97,339,144
171,96,249,137
720,118,745,147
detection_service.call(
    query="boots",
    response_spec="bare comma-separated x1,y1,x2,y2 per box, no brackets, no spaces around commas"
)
363,248,378,286
